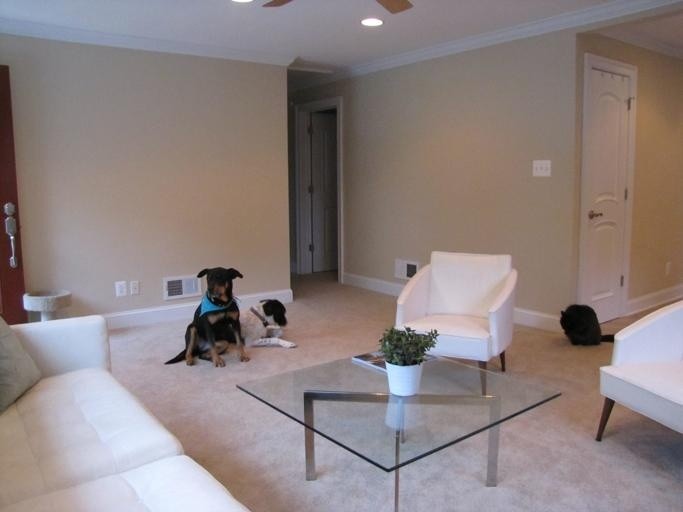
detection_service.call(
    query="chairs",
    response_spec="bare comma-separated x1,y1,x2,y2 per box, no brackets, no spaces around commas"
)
395,264,519,396
596,300,683,441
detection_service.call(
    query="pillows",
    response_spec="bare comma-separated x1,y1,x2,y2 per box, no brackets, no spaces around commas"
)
0,315,42,415
427,251,512,319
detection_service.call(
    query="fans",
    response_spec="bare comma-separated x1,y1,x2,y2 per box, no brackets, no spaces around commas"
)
262,0,414,14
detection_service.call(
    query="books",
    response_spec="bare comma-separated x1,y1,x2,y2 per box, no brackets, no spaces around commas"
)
350,346,434,372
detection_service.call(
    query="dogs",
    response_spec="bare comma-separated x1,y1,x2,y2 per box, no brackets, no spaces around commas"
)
239,299,297,347
164,267,250,367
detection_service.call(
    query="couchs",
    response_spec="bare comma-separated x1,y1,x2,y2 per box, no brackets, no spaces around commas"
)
0,314,251,511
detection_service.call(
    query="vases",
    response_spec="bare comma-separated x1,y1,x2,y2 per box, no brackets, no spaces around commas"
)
384,394,427,430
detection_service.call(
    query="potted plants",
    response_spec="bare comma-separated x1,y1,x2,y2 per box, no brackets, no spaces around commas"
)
377,325,440,396
